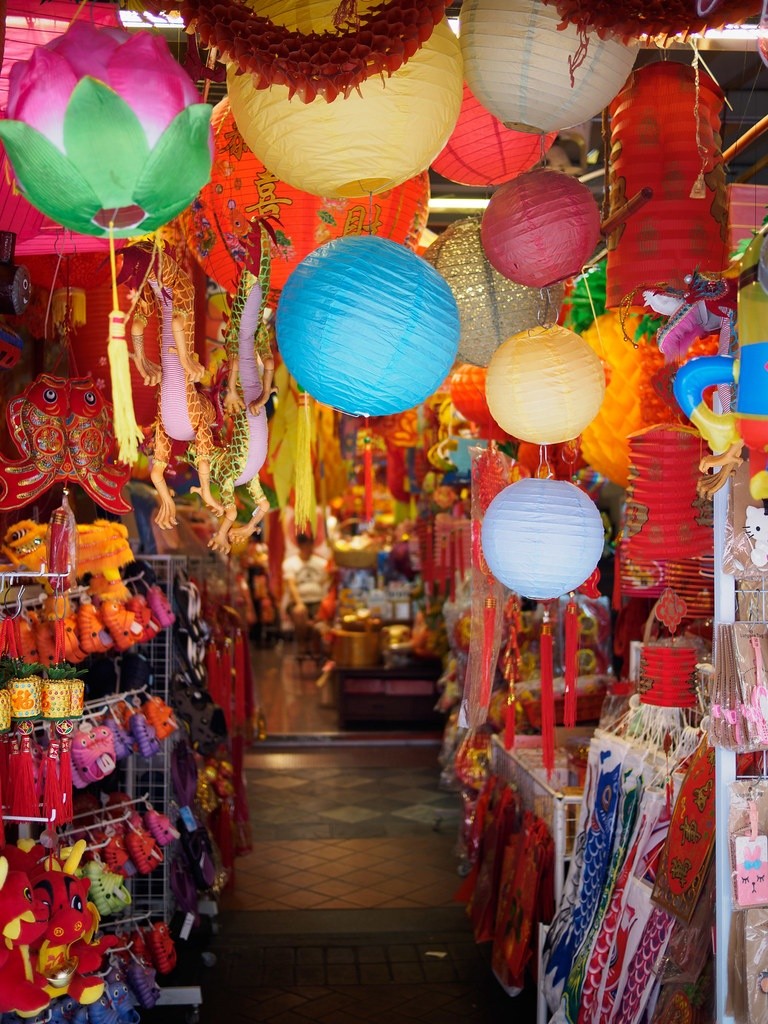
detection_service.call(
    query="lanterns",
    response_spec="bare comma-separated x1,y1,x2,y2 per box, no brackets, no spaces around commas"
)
0,0,564,443
459,0,768,705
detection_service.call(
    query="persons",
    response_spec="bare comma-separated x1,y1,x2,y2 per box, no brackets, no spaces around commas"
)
282,530,329,660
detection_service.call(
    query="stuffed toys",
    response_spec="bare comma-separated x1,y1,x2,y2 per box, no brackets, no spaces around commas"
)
1,519,136,620
0,838,120,1018
